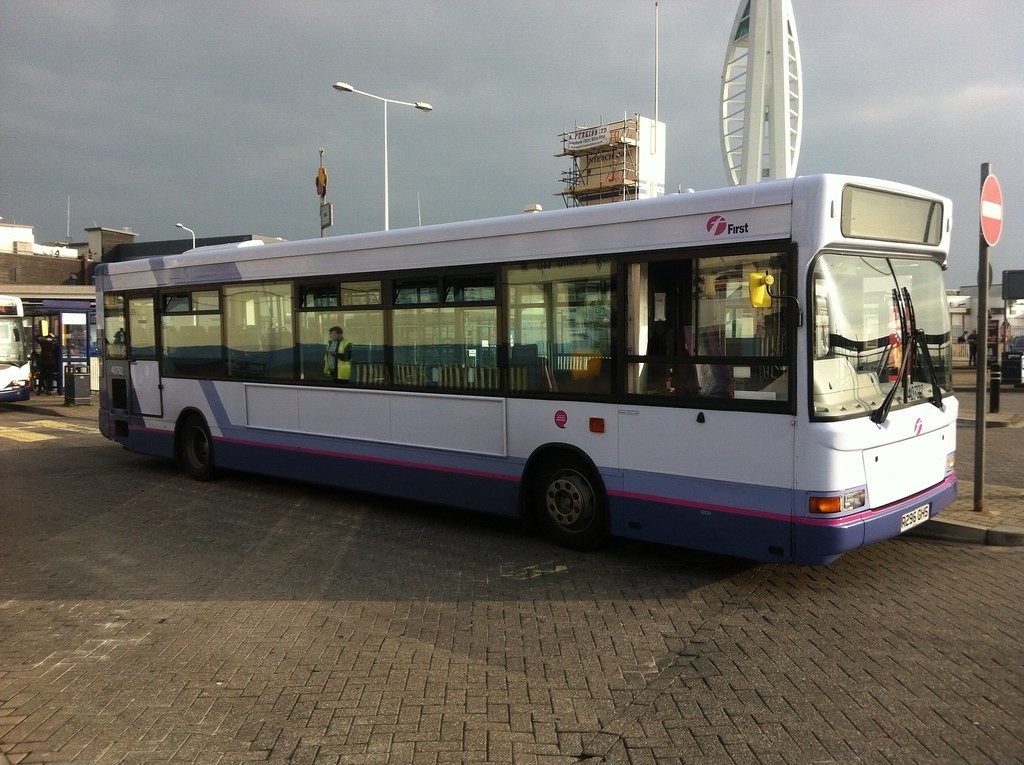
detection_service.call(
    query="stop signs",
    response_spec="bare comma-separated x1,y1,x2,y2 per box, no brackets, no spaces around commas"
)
979,174,1003,245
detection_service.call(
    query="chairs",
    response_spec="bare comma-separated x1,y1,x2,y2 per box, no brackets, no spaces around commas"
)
512,345,557,393
684,322,737,396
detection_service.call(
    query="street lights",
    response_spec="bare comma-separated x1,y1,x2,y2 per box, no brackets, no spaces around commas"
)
331,81,434,232
175,222,197,326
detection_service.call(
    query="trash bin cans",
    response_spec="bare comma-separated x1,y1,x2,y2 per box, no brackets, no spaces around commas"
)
64,363,93,406
1000,351,1024,384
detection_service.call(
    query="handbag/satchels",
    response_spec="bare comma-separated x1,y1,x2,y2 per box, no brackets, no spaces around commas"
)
958,337,966,343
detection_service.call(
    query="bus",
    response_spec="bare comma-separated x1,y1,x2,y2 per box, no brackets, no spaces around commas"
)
0,295,32,404
95,174,956,565
